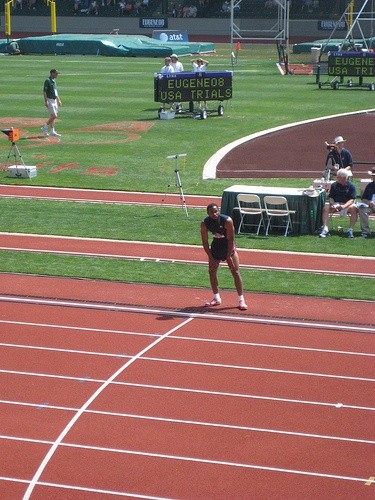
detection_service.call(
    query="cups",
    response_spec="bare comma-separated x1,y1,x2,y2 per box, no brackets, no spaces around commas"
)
334,164,339,170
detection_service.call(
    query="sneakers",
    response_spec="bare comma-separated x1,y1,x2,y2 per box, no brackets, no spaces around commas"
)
50,132,61,136
204,298,221,306
363,232,370,238
239,300,248,309
347,230,354,238
41,128,49,136
320,230,329,238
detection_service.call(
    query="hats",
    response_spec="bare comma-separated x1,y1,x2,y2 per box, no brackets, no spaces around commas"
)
170,54,178,59
337,168,349,177
368,168,375,176
335,136,346,144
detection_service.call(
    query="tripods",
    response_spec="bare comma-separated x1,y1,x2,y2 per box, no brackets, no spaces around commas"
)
3,142,32,182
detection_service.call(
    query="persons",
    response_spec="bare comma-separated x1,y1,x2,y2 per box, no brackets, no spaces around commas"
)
39,69,64,138
189,58,209,110
318,169,360,238
357,166,375,238
169,54,184,109
200,203,250,311
159,57,174,112
11,1,319,18
324,135,353,172
